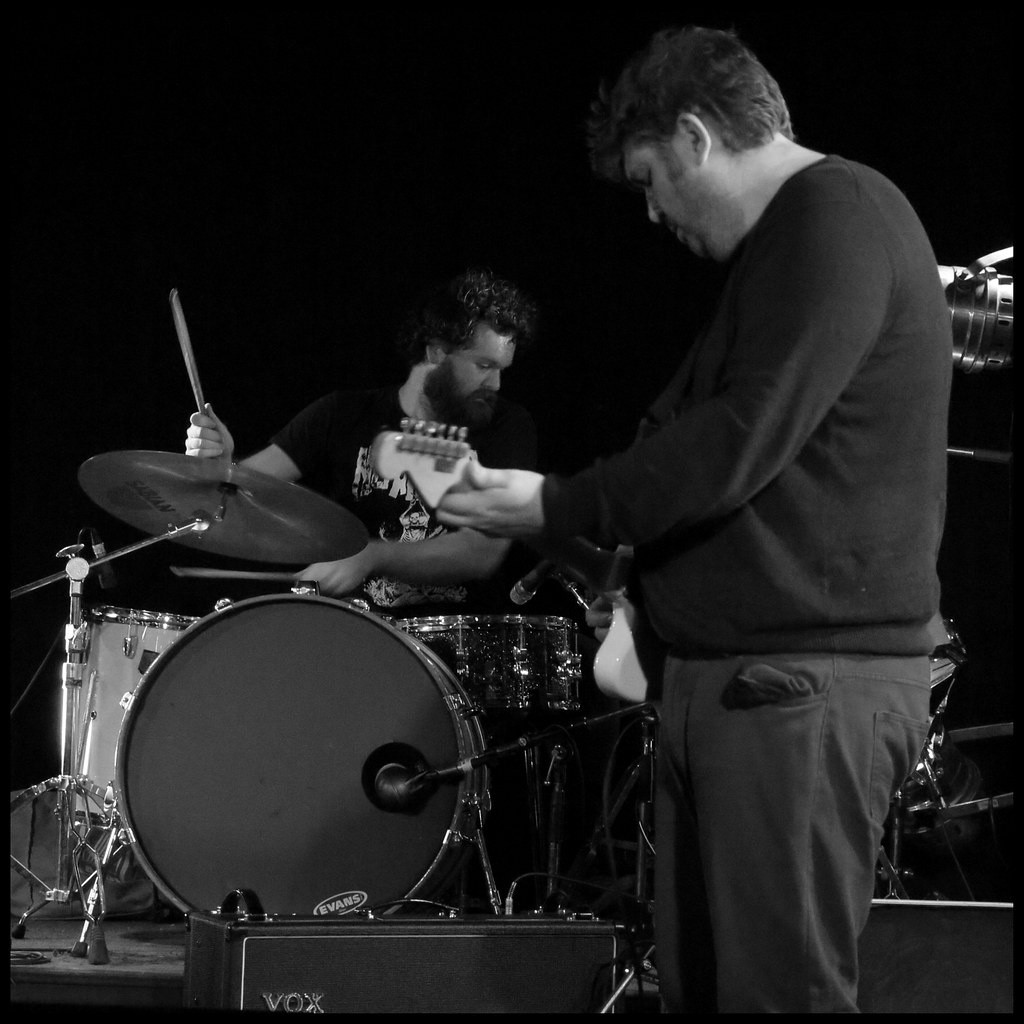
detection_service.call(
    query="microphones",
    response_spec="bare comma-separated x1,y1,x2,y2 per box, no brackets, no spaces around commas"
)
510,559,556,605
374,764,411,803
90,526,116,588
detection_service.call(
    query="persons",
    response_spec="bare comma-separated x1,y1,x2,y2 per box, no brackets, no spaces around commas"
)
185,269,536,914
435,28,950,1024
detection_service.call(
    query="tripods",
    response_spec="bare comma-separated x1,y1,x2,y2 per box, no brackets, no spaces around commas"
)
555,706,660,926
11,497,226,942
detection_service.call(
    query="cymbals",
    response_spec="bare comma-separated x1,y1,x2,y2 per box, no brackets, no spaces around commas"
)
78,447,369,567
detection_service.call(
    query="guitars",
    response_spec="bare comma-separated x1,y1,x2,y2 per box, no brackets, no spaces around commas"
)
369,420,667,664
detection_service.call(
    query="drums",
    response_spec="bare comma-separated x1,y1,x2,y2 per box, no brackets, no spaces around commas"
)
108,588,491,929
70,603,208,829
387,609,586,717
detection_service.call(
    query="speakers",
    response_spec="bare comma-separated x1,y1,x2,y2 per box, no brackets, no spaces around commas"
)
852,899,1015,1015
184,907,625,1016
